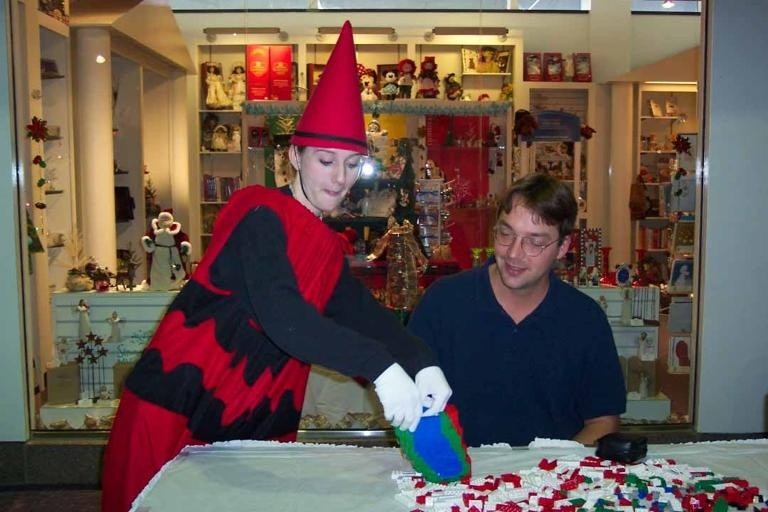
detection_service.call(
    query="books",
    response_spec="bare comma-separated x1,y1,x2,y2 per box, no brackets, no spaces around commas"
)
631,96,697,375
462,47,512,74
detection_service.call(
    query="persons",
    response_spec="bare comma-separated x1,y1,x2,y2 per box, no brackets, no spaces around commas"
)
360,56,463,101
102,143,454,511
405,173,626,448
106,311,120,343
75,299,91,341
203,63,246,152
140,211,191,291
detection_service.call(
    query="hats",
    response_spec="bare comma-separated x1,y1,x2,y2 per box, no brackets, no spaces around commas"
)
290,20,369,153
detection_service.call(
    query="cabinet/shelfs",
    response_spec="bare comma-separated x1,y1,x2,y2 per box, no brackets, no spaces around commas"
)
190,35,699,315
39,25,145,290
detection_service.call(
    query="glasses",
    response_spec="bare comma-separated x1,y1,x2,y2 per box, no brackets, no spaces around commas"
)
493,224,564,256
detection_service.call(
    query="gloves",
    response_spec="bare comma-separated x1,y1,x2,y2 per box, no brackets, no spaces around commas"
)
415,365,453,418
375,362,421,432
